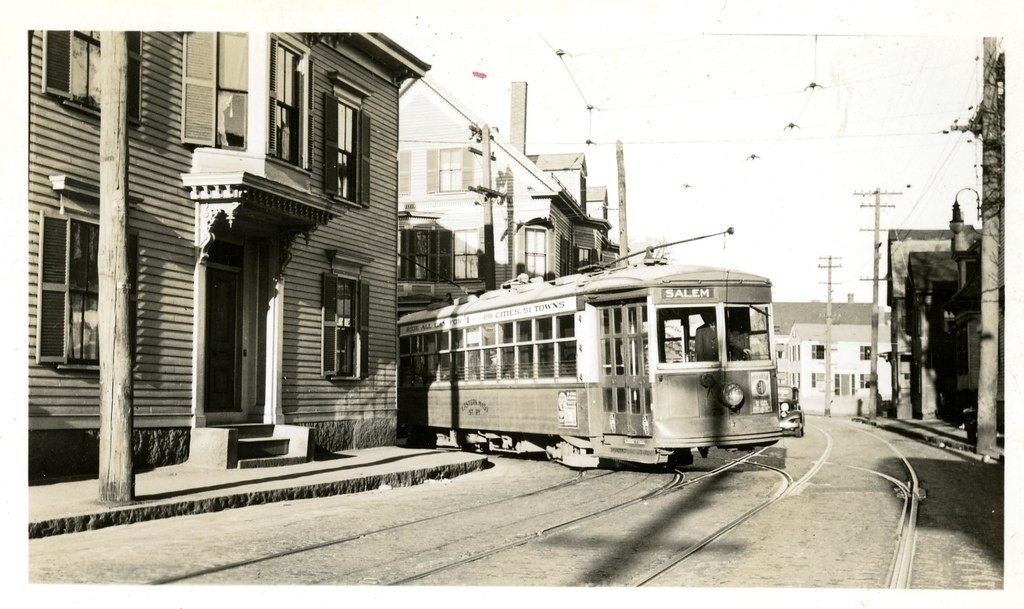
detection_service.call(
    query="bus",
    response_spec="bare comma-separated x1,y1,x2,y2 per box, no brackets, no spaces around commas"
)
398,249,783,469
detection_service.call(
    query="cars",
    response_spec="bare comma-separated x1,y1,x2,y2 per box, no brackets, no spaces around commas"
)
778,384,805,437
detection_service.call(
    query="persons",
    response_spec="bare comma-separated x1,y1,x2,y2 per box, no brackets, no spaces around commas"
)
695,310,752,362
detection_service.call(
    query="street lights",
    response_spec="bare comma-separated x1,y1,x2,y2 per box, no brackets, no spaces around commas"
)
949,188,999,452
